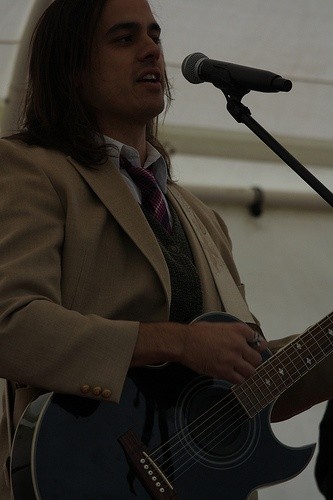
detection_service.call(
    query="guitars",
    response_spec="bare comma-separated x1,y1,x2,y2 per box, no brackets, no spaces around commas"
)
10,305,333,500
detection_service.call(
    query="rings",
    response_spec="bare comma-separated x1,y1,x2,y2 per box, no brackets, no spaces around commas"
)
249,331,263,347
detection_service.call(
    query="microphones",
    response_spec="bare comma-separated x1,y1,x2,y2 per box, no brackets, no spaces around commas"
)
181,52,293,93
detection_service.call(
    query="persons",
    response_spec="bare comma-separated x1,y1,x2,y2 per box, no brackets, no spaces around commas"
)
0,0,304,500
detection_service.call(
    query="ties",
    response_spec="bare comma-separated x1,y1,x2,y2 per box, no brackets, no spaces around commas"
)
120,158,171,234
